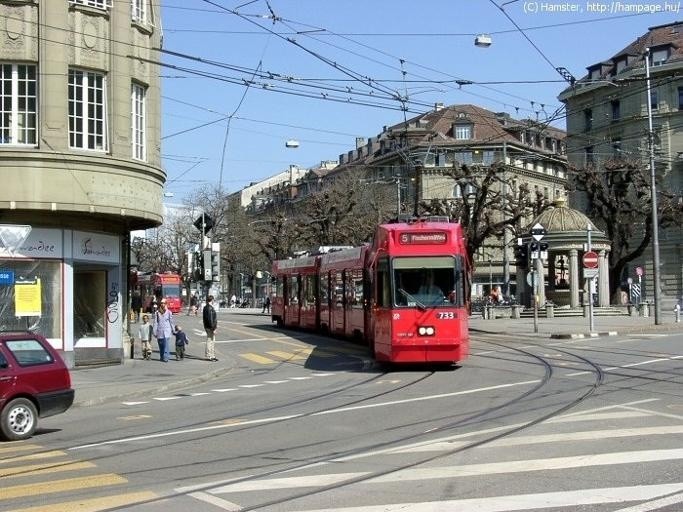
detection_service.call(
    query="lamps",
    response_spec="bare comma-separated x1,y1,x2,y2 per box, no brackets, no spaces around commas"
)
474,33,492,48
286,137,299,148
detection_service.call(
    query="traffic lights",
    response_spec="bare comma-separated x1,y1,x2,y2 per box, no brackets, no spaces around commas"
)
203,250,217,282
514,244,525,269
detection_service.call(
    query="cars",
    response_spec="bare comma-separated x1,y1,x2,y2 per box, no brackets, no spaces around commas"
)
1,332,73,440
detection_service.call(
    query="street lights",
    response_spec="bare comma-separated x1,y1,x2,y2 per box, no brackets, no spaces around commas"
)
642,49,661,326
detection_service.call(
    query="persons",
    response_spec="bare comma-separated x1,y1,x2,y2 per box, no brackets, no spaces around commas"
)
203,295,218,361
152,299,175,362
229,293,249,309
187,294,197,316
173,325,189,361
491,285,499,303
261,292,270,314
138,315,154,360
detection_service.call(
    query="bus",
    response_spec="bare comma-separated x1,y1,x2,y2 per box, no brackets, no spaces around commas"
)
268,217,470,372
132,272,182,317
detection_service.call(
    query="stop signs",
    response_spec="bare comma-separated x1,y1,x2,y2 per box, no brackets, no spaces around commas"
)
582,251,599,270
